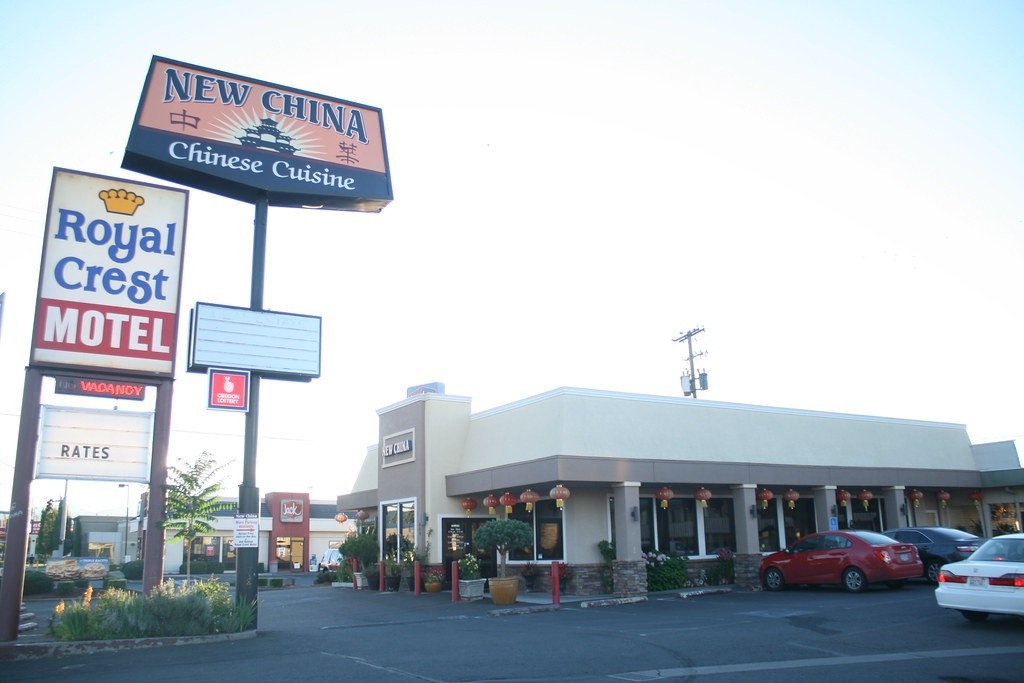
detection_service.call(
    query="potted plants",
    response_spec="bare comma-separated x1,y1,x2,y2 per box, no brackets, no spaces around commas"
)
474,518,534,604
403,551,420,591
383,558,402,592
338,535,380,591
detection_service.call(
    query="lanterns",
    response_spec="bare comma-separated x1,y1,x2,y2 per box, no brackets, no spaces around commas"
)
908,488,923,507
550,485,570,507
784,488,800,508
836,490,850,507
482,494,499,515
857,489,872,507
500,492,518,513
694,487,711,508
335,513,347,526
460,497,477,516
968,490,982,506
655,487,673,508
756,488,774,508
356,509,369,524
936,489,950,507
519,489,540,511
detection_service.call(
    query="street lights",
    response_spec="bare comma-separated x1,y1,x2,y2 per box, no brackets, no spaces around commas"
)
119,484,129,555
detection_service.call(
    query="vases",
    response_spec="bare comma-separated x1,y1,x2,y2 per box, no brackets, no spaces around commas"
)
559,577,568,595
524,575,537,592
459,578,486,601
424,581,442,592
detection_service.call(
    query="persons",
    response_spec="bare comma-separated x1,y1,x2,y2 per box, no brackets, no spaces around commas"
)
28,554,35,570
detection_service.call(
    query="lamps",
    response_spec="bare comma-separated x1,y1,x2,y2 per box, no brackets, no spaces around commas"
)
832,505,837,517
749,505,757,518
901,504,906,514
631,507,639,521
421,512,428,526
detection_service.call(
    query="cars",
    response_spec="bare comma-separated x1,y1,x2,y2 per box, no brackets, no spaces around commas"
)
934,533,1024,622
105,571,127,590
318,549,343,572
883,528,985,584
758,530,924,594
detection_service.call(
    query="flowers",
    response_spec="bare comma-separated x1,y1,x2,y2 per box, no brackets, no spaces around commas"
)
550,562,572,580
420,566,447,583
519,561,542,578
458,553,481,580
